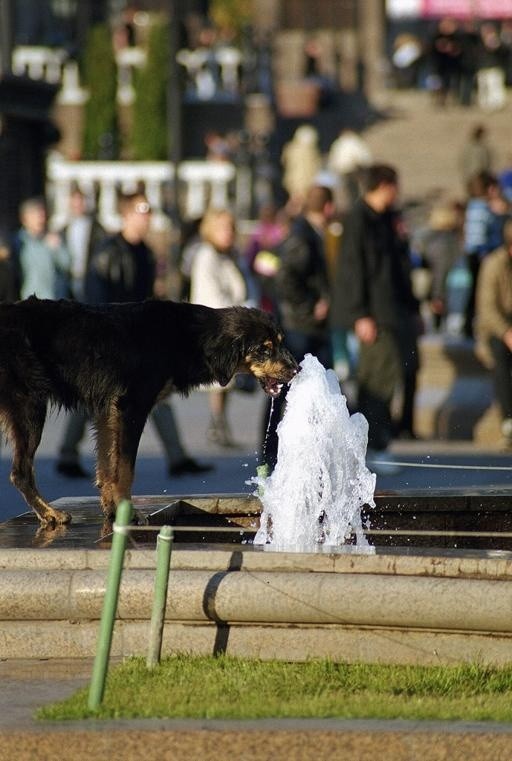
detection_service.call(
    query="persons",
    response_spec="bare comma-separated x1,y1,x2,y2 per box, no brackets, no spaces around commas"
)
2,1,512,482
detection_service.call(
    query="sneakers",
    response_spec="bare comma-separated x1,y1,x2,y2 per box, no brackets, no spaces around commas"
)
362,449,403,478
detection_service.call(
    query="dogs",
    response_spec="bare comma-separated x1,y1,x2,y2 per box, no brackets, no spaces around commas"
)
1,293,302,542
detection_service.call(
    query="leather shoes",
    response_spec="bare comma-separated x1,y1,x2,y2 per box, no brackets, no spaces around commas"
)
165,454,216,479
54,455,93,481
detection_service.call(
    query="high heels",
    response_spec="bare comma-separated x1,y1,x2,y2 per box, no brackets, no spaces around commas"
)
203,411,244,451
